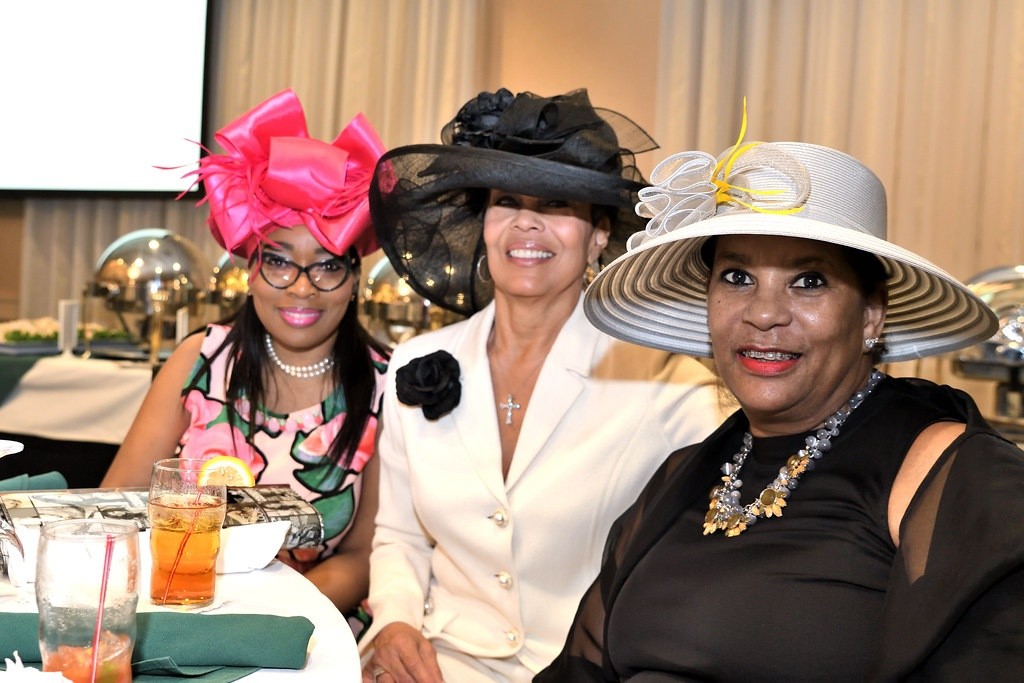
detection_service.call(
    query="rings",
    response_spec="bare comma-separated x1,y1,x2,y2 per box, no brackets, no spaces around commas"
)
372,667,385,683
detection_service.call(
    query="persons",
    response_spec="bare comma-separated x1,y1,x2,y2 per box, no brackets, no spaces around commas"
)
356,185,743,683
103,226,403,615
532,143,1024,683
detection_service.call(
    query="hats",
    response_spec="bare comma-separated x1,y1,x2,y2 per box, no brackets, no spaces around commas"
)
152,88,401,259
583,94,999,364
368,87,661,317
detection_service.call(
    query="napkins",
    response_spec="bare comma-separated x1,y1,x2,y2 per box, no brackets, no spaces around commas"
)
0,612,315,683
6,519,291,588
0,472,68,492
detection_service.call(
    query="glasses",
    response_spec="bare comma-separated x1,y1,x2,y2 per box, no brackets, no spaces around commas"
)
251,251,352,292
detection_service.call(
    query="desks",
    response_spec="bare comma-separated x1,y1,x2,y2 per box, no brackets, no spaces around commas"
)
0,352,161,488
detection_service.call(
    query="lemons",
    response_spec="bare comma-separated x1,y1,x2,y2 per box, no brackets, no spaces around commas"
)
197,456,256,487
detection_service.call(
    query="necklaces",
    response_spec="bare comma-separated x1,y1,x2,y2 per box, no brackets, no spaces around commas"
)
493,347,544,425
703,370,885,536
265,335,335,377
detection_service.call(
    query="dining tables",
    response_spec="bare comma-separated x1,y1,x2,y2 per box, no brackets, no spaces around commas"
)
0,492,363,683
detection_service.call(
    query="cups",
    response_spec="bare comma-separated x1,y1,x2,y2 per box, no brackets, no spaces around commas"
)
147,458,227,611
34,518,141,683
